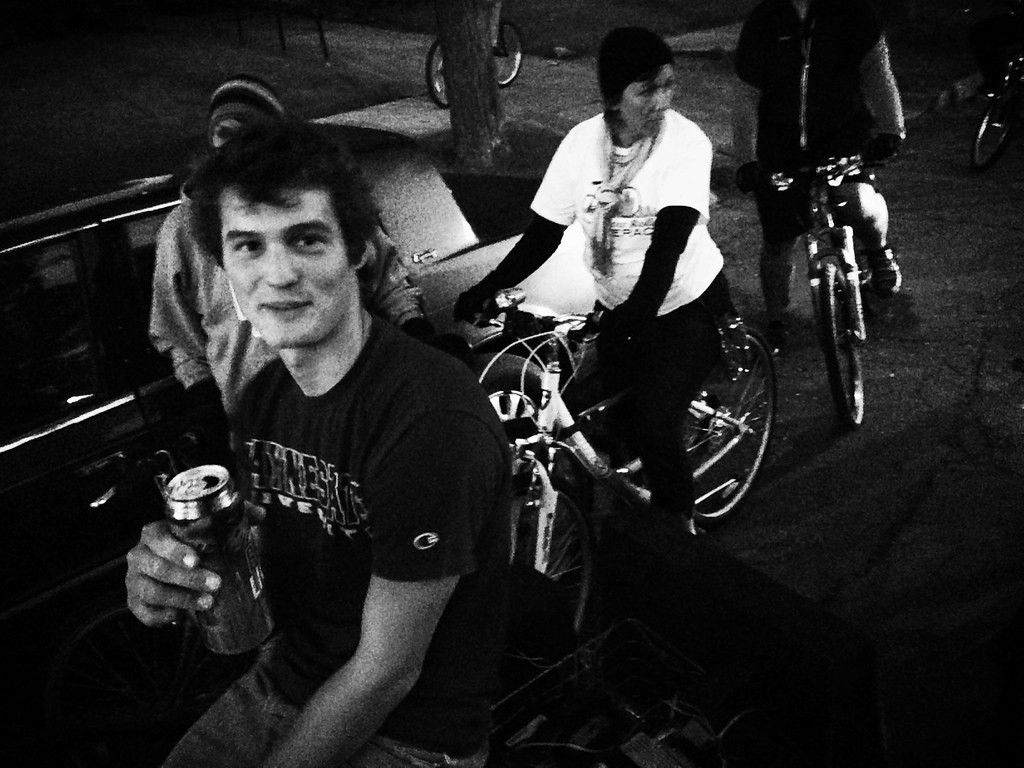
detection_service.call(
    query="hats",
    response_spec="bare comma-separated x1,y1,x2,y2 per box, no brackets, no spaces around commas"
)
207,75,287,130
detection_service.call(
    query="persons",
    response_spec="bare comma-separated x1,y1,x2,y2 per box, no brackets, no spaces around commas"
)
453,26,734,520
148,72,434,415
725,0,906,349
125,121,514,768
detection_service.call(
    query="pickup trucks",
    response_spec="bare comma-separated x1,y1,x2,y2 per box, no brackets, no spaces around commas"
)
1,122,720,768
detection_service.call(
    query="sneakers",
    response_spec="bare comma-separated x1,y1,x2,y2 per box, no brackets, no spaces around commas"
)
764,317,791,347
861,246,908,297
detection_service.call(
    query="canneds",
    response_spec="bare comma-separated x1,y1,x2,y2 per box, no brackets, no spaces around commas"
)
163,465,273,656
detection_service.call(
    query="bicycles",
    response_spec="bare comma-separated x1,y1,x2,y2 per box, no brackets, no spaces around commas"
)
963,7,1024,173
462,286,778,695
483,616,765,768
755,146,898,431
425,20,526,109
